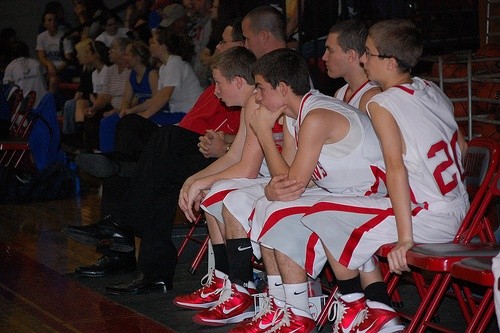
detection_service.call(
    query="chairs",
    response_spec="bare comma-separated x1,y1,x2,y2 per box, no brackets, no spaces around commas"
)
177,137,500,333
0,85,55,170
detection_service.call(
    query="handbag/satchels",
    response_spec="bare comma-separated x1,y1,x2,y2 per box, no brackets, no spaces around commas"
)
4,172,38,204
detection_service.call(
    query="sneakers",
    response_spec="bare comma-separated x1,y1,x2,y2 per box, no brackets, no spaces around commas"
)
258,305,319,333
174,269,228,309
327,293,367,333
193,276,255,326
348,300,405,333
226,294,284,333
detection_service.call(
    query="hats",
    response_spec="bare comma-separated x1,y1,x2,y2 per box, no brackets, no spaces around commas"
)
159,4,185,28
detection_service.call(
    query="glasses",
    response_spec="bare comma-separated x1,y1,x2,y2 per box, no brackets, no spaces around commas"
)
365,49,390,59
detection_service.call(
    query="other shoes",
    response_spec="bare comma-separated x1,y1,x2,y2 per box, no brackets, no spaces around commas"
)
75,152,143,178
104,271,172,296
61,214,135,253
74,251,136,278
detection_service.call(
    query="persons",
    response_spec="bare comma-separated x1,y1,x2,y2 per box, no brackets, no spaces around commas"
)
0,0,205,296
178,0,388,332
301,20,471,333
